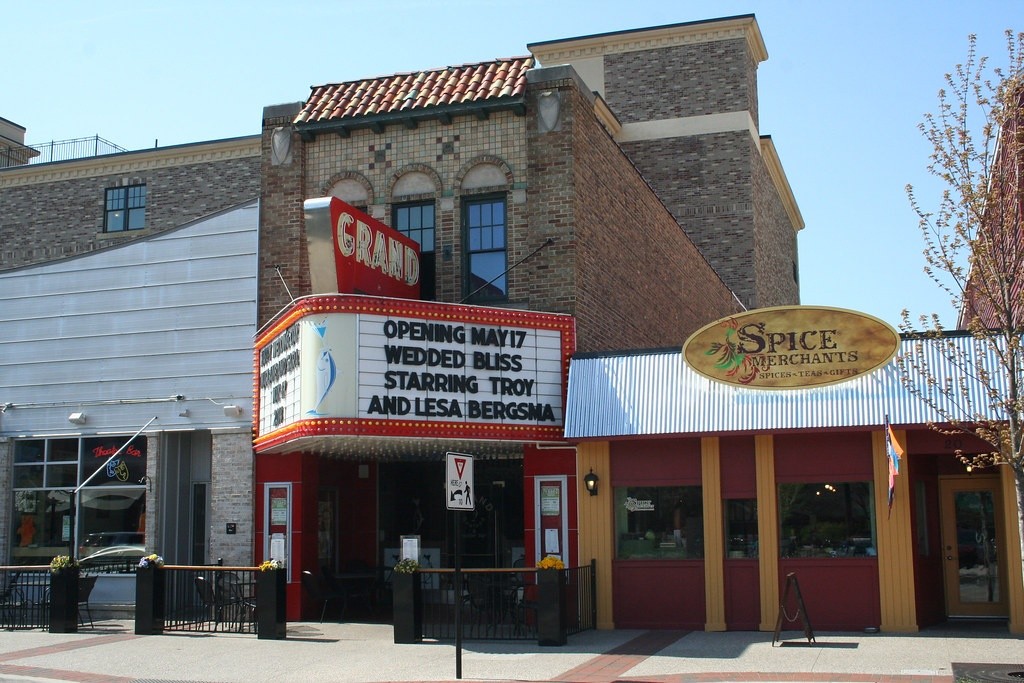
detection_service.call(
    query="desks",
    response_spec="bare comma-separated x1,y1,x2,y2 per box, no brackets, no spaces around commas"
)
335,573,378,617
11,581,50,627
225,580,256,628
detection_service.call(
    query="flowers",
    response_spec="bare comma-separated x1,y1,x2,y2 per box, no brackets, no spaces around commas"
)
259,560,280,572
139,554,164,568
536,557,565,570
394,558,419,573
50,555,79,572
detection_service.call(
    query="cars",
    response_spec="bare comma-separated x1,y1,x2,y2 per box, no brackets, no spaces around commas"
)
79,532,145,558
959,564,992,583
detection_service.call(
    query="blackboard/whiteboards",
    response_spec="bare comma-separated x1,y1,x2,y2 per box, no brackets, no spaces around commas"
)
776,572,813,639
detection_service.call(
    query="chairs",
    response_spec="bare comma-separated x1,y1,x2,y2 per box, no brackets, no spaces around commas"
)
447,559,538,638
195,572,256,635
0,570,98,630
302,565,394,622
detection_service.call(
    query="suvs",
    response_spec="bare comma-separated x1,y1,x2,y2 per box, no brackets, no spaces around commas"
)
78,546,145,572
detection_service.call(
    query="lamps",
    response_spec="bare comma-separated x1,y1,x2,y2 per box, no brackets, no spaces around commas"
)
69,413,86,424
223,405,240,416
584,467,599,495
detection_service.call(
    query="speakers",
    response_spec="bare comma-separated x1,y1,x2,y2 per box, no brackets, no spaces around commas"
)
224,406,240,417
69,413,85,424
